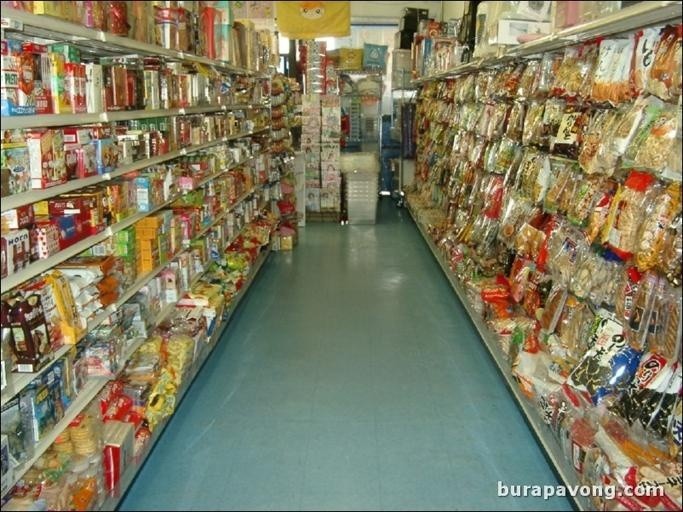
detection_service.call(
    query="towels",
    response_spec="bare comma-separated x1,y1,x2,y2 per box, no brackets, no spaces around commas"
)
274,3,350,40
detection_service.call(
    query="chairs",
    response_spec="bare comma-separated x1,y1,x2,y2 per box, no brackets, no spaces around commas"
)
396,1,681,511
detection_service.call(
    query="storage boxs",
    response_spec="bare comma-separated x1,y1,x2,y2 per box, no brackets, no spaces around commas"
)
341,171,379,225
392,6,429,89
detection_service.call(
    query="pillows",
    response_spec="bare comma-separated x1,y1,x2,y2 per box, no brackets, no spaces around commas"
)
362,43,386,70
339,47,362,70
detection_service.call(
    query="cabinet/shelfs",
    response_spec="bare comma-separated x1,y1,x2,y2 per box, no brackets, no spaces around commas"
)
1,0,302,512
334,71,383,156
